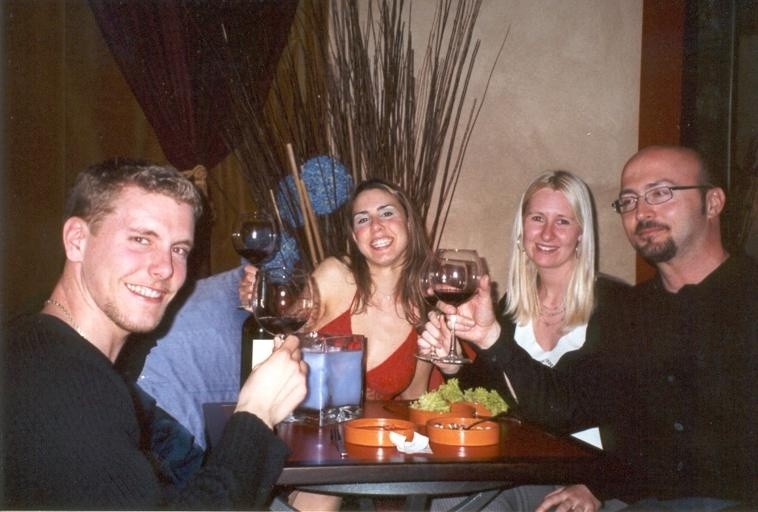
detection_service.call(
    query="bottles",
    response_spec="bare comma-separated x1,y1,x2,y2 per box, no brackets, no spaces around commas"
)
239,282,288,390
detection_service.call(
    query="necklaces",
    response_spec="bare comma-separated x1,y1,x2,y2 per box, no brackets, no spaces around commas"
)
540,304,565,318
378,291,394,299
44,299,83,337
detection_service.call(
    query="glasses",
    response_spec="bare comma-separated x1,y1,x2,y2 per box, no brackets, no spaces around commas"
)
612,183,708,215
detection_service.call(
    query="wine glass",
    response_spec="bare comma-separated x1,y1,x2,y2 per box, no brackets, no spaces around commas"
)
412,247,483,367
250,264,314,424
230,211,278,314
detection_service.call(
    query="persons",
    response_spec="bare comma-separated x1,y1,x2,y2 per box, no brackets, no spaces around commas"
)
0,156,310,512
426,144,758,512
240,180,434,512
417,171,635,512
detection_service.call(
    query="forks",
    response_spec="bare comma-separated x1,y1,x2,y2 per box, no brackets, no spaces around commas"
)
329,428,349,458
